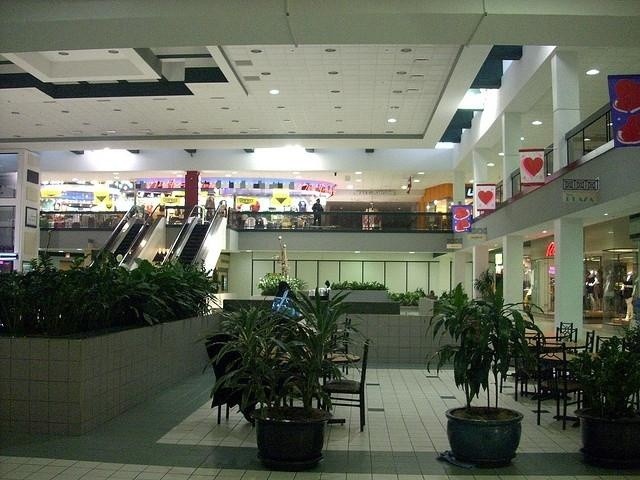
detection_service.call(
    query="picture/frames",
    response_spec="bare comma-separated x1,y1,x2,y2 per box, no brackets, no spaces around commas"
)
25,206,38,229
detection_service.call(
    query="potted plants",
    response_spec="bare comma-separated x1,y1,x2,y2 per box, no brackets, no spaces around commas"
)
565,320,639,470
195,287,365,471
422,266,550,470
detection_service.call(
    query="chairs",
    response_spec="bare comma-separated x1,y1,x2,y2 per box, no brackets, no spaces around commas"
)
204,318,373,436
492,318,640,434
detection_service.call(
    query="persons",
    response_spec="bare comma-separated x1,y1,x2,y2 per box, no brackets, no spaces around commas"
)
312,198,324,231
276,280,300,325
585,269,599,312
621,271,636,322
324,279,331,297
426,290,438,300
